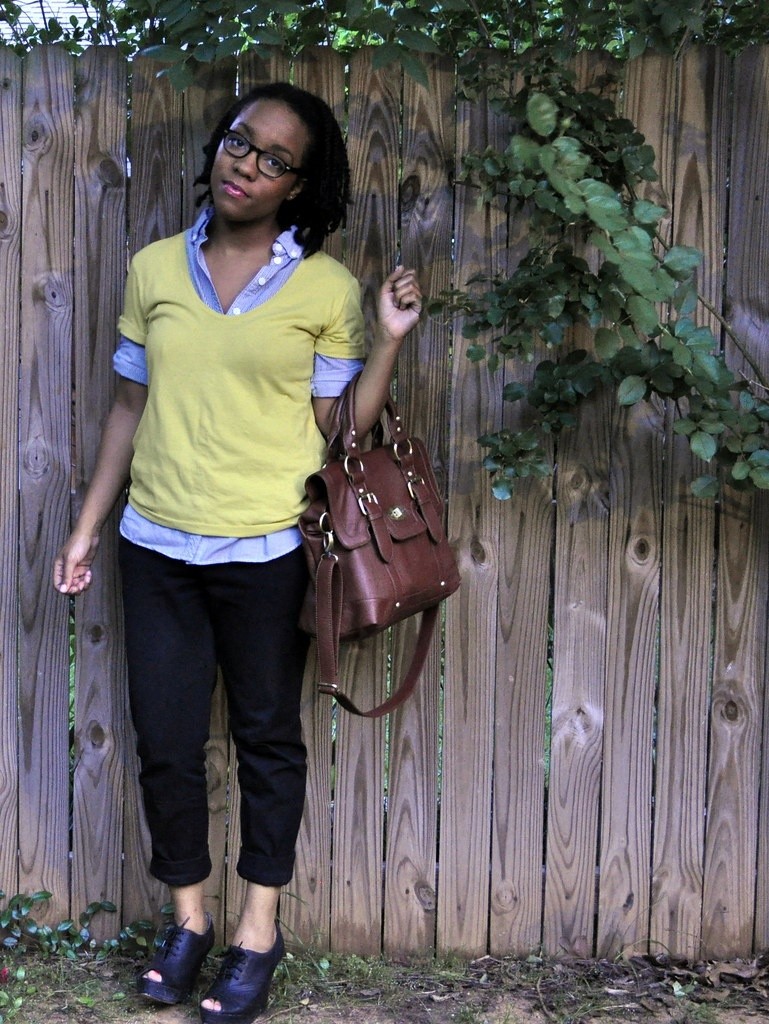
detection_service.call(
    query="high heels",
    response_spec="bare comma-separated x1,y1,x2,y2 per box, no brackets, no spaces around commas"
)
136,912,215,1005
200,923,284,1024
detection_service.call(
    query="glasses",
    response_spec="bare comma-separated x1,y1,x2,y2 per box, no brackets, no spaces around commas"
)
222,128,300,178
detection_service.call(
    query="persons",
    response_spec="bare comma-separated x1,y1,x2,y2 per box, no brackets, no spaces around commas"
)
52,82,422,1024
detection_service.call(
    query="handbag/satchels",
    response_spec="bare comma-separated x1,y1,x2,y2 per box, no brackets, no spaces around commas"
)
295,368,461,718
